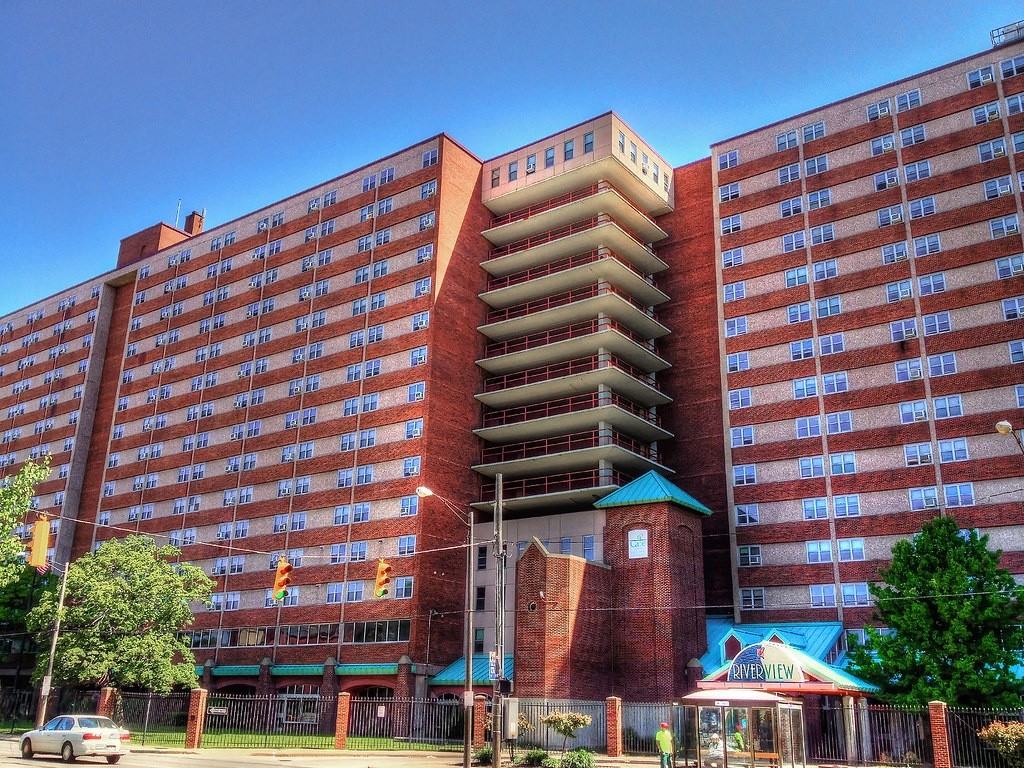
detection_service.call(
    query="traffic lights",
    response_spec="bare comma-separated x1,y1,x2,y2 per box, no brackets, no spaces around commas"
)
273,556,294,604
500,680,513,694
373,557,393,598
24,521,49,565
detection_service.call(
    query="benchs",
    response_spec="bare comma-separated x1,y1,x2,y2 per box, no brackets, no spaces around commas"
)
693,748,778,767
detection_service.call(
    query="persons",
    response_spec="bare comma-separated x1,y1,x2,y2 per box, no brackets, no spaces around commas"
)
656,723,673,768
704,734,723,768
734,726,746,752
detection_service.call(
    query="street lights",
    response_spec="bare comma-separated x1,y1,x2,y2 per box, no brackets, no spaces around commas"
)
414,482,475,767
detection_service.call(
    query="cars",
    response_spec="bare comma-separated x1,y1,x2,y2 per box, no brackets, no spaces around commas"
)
19,714,132,764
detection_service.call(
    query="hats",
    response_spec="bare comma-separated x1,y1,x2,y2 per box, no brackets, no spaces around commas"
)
735,725,744,730
660,723,670,728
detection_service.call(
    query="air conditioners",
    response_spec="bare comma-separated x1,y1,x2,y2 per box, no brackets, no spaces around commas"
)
642,163,649,173
750,557,760,563
526,164,534,174
0,185,434,610
877,73,1024,507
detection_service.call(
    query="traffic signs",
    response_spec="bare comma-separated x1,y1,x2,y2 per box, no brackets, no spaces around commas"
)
207,707,229,715
